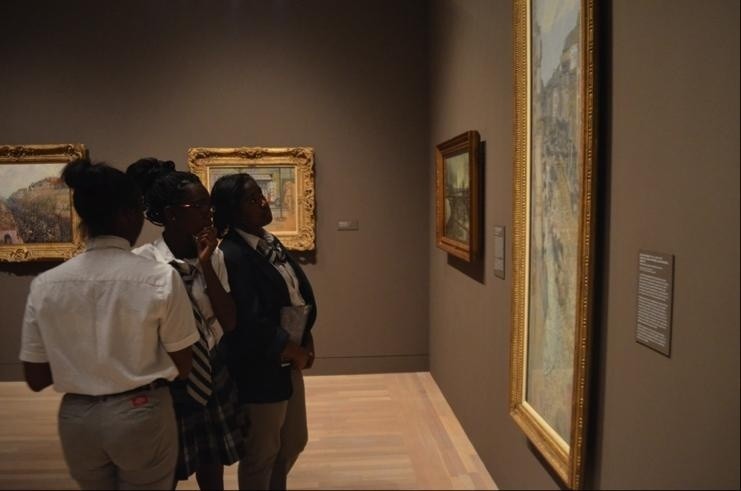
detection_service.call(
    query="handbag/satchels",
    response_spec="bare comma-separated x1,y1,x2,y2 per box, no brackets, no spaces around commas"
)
278,304,312,346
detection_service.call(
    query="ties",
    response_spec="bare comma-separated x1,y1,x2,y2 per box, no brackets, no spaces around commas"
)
170,262,213,406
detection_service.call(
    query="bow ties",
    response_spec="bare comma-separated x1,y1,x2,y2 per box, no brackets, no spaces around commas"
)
257,241,288,265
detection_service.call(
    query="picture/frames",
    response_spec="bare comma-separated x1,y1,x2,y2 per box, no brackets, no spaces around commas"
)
506,0,606,491
1,143,89,266
185,145,319,255
434,130,486,267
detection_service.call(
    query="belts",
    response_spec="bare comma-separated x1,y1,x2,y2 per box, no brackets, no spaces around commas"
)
112,376,169,396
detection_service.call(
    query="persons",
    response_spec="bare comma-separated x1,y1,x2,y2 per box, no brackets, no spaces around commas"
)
20,157,201,491
210,173,317,491
126,157,245,491
